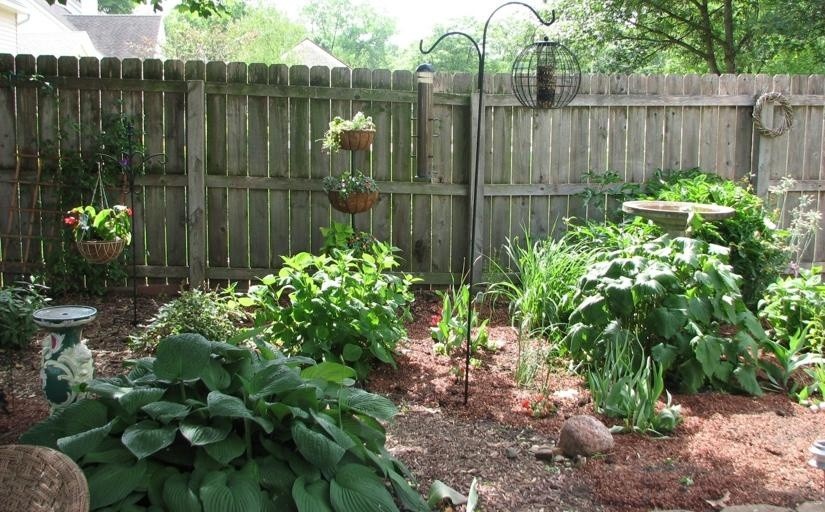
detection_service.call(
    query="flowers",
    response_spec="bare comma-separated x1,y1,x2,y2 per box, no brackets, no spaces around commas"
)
64,205,133,247
321,167,378,200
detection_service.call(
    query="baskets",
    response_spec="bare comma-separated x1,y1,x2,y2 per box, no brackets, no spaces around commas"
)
328,190,379,214
341,129,377,151
77,239,126,263
0,444,91,512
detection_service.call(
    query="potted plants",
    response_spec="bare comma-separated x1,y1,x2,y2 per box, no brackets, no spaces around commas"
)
319,112,375,159
